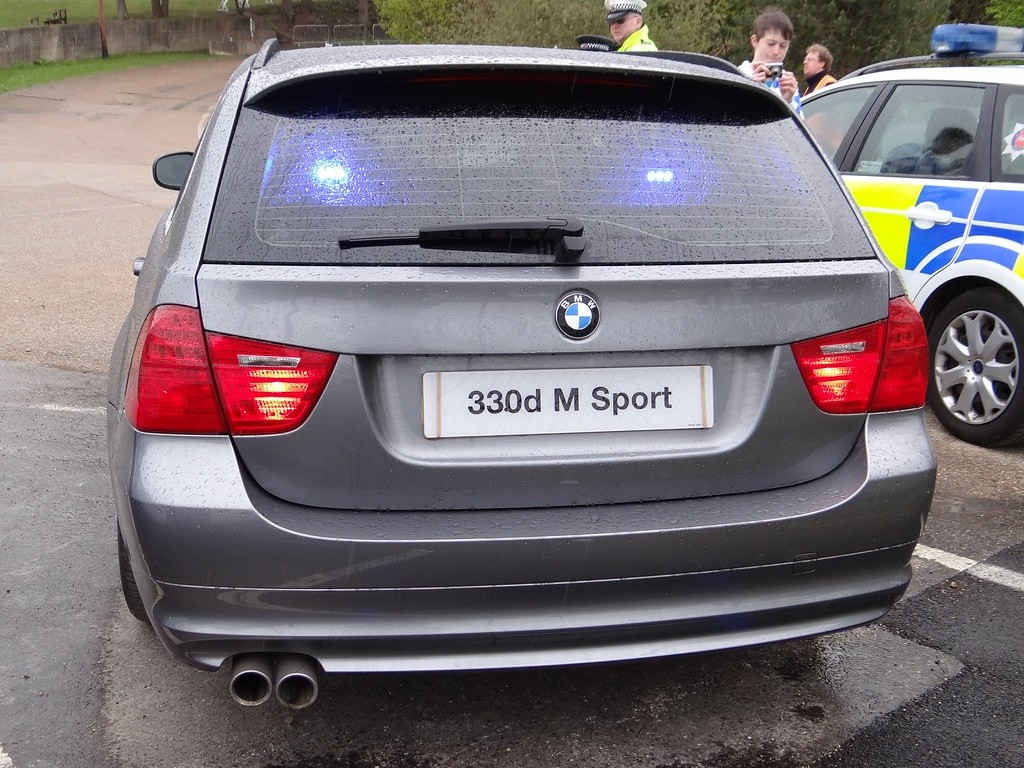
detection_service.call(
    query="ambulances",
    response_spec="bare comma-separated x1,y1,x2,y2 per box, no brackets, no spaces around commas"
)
799,24,1024,446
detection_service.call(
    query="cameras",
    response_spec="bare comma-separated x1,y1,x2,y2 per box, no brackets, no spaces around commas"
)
762,62,783,79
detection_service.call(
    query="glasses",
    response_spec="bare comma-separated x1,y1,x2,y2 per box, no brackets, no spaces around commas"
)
610,16,638,25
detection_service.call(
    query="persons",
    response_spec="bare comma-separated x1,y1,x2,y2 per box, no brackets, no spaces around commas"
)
737,5,805,119
604,0,657,52
800,42,838,96
878,106,978,176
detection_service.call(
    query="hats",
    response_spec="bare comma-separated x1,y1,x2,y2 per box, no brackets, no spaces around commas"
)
605,0,648,20
575,34,613,52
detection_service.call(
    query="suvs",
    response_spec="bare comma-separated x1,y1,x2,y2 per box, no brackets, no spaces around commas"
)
105,36,938,711
